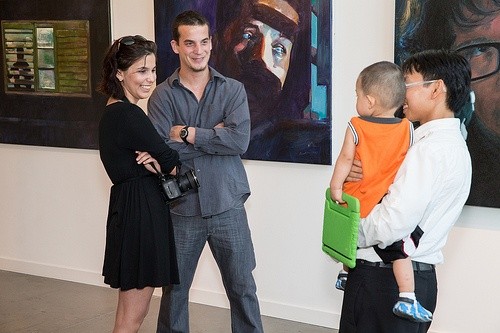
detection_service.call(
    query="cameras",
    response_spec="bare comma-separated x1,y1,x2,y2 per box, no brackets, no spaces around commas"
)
157,168,201,206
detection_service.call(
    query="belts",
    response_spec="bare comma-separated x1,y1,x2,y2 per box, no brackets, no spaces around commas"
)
356,259,435,272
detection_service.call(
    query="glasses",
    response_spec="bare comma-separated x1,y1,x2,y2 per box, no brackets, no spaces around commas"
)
452,42,500,82
404,79,447,94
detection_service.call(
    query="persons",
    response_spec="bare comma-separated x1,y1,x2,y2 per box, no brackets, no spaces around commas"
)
147,14,265,333
330,62,435,324
98,35,183,333
337,50,473,333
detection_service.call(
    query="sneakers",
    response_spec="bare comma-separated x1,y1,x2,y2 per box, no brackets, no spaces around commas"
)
392,297,432,322
335,273,348,291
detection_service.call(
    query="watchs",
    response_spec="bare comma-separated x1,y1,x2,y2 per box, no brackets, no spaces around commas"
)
180,125,192,144
